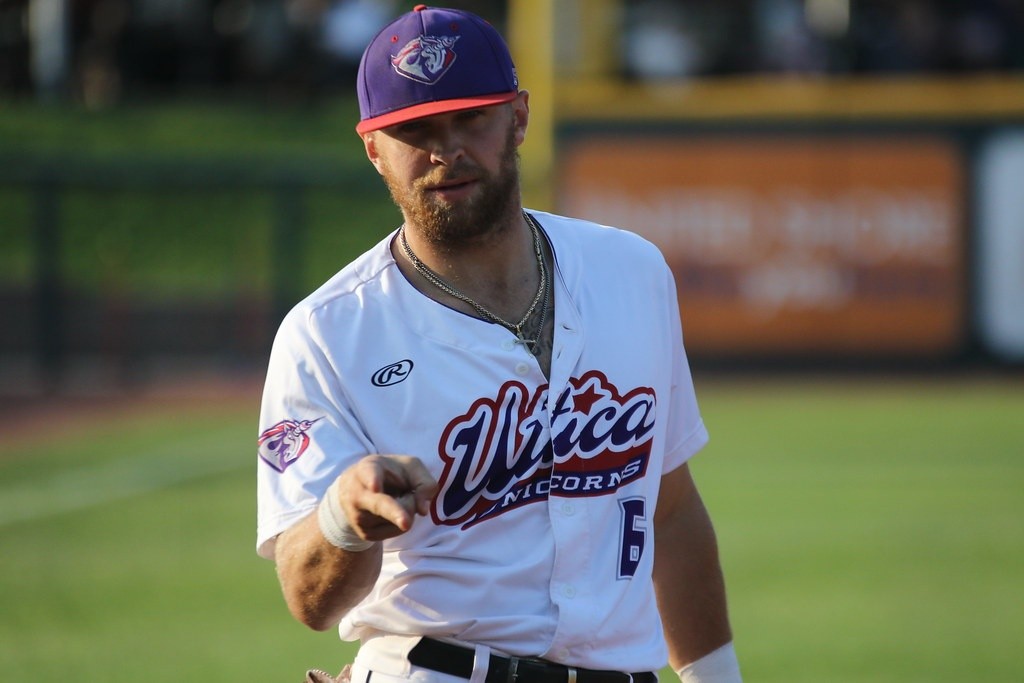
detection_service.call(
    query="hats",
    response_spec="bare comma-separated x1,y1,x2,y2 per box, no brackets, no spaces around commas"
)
355,4,518,133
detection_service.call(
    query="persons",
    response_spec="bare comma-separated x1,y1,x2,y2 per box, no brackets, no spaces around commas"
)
256,8,744,683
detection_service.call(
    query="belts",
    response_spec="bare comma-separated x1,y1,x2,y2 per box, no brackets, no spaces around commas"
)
407,636,659,683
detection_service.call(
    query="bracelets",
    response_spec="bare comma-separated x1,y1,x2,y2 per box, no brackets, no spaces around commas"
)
676,639,743,683
319,478,374,550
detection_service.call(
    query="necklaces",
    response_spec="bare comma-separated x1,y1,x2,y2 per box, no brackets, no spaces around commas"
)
400,207,549,356
